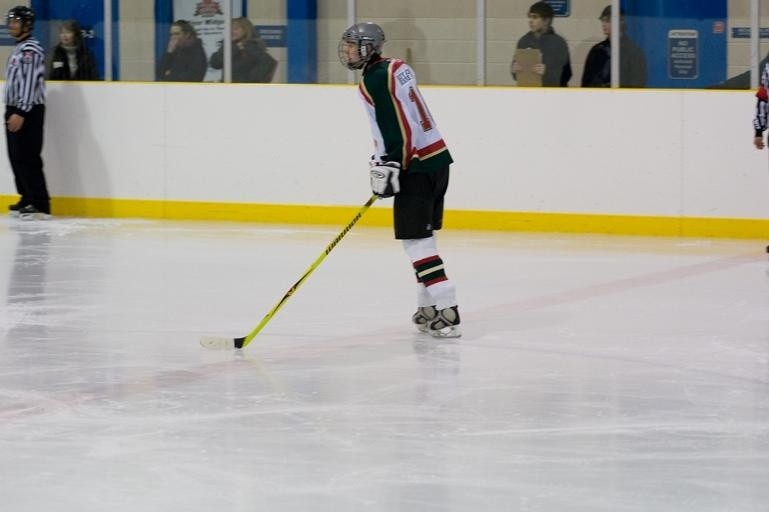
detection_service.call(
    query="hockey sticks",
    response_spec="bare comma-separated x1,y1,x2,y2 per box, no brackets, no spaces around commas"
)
201,193,382,351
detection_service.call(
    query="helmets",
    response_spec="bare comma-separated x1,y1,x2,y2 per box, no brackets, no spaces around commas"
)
336,22,386,71
6,5,34,39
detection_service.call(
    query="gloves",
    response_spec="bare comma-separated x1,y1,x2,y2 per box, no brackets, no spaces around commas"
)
369,157,400,198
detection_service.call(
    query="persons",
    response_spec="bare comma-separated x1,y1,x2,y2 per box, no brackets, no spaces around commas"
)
752,61,769,255
510,3,573,87
338,21,460,331
580,5,646,88
209,16,278,83
3,7,54,215
704,51,769,89
49,20,98,80
157,20,207,82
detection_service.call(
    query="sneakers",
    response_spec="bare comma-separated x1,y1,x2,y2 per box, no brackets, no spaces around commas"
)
424,305,461,330
8,199,27,210
20,204,51,213
412,304,438,326
